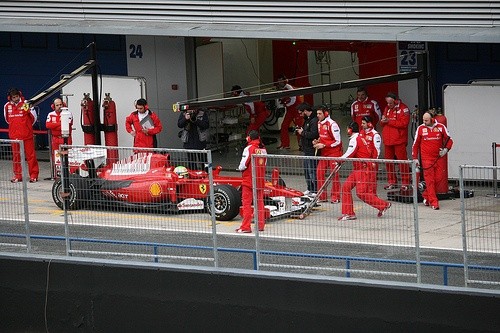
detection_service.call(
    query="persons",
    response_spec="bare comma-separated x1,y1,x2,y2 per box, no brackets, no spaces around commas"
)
125,98,162,153
412,112,453,210
232,91,270,147
296,102,322,196
278,74,303,150
46,98,74,179
380,95,410,191
332,122,392,221
312,105,343,203
351,88,381,133
4,88,39,182
236,130,267,233
361,116,382,196
178,108,209,173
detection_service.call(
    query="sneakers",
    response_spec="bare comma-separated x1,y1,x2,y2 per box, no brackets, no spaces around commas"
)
423,198,429,205
384,184,398,190
432,206,439,210
401,185,408,191
29,178,37,182
11,178,22,183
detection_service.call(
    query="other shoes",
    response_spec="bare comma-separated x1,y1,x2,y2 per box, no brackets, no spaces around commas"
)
304,190,310,195
236,228,251,233
331,199,340,204
378,202,391,217
276,146,289,150
316,199,327,203
311,193,318,197
338,213,355,220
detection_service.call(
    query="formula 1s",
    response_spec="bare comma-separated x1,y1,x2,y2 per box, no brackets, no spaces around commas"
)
51,148,318,221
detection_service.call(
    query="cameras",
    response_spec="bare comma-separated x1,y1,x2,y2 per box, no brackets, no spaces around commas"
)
292,126,300,135
189,113,196,121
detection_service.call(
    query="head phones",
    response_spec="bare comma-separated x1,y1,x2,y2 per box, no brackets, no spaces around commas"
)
347,122,353,135
51,98,66,110
246,132,261,141
135,99,148,109
322,104,328,117
392,95,399,104
6,90,22,100
429,112,435,124
365,115,372,127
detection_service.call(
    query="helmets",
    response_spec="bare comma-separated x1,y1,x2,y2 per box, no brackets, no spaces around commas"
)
175,166,189,179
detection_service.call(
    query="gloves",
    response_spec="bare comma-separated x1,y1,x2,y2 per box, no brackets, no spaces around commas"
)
413,159,421,173
439,147,448,157
